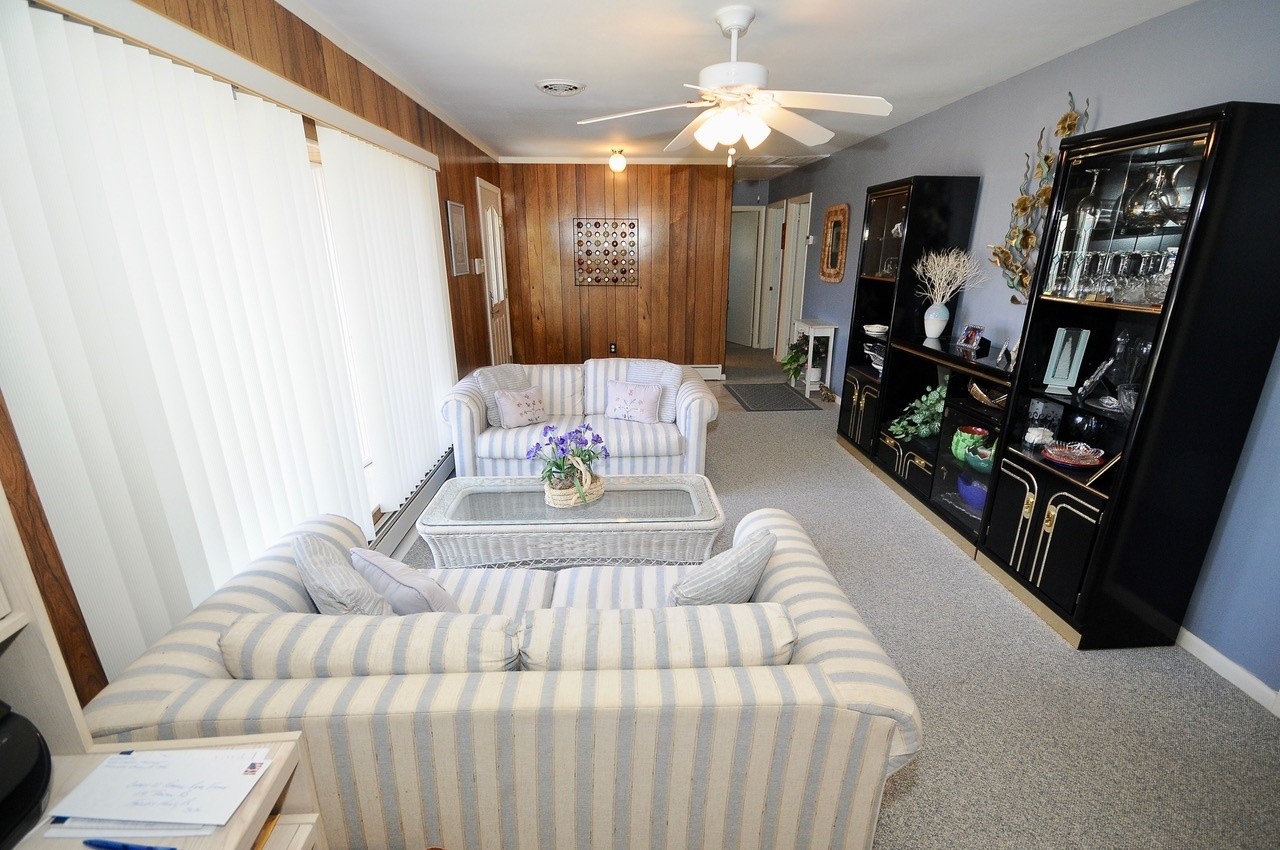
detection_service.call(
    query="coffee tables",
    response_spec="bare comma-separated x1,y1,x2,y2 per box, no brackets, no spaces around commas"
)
415,474,726,572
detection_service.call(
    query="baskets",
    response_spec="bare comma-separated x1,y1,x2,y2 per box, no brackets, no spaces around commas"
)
544,454,604,508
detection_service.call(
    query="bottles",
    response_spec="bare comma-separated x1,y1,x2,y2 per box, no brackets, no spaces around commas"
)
1098,327,1152,410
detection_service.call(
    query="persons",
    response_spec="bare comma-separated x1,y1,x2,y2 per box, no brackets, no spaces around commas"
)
963,330,975,346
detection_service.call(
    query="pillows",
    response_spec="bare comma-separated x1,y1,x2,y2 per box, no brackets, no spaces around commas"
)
473,363,529,427
351,547,463,616
668,527,777,606
605,380,662,424
291,534,396,616
627,359,683,423
494,385,548,429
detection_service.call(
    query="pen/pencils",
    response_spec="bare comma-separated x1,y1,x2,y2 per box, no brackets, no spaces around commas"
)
83,839,178,850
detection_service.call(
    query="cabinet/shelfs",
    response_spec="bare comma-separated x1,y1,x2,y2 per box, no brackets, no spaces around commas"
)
789,318,839,398
836,101,1280,650
0,390,333,850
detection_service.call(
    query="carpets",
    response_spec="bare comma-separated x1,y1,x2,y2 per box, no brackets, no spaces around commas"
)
723,383,824,411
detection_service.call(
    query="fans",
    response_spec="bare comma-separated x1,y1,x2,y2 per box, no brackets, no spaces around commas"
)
577,4,893,146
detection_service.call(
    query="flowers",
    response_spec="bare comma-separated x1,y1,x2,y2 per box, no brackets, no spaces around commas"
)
781,330,826,383
988,92,1089,306
526,424,610,510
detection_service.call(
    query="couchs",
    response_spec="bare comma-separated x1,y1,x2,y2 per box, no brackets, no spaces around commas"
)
82,508,925,850
442,358,719,478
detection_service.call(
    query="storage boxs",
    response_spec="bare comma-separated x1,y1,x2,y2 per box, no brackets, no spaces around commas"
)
1021,398,1065,454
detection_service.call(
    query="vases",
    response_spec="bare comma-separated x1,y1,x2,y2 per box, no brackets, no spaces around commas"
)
924,303,949,339
806,368,822,382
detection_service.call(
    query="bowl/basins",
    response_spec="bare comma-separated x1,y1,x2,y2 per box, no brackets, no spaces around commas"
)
966,445,993,474
951,426,989,462
1044,440,1105,463
1117,384,1141,422
1163,204,1190,226
958,475,986,509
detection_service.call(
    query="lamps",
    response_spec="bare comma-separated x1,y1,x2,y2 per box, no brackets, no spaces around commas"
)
693,96,769,168
609,150,627,172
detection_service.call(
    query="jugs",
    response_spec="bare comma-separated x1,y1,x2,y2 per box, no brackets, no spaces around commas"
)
1124,165,1170,227
1157,164,1184,226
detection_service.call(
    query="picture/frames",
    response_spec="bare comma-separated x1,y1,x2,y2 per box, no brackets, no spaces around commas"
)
956,324,985,349
446,199,470,277
997,338,1009,365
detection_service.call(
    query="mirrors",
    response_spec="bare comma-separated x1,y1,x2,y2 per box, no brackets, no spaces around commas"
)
819,204,850,283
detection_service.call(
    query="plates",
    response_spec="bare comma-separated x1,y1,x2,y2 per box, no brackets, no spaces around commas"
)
863,324,889,333
1086,398,1122,412
1041,447,1104,468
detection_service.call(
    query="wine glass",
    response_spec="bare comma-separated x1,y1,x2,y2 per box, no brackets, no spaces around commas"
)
1075,168,1111,230
1042,247,1173,308
